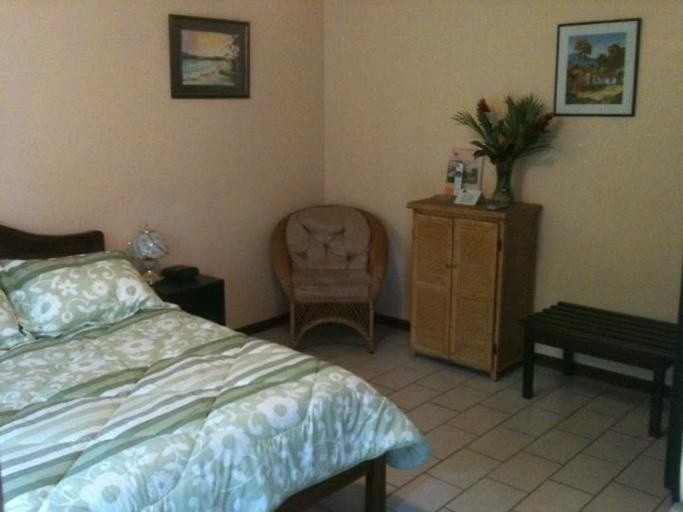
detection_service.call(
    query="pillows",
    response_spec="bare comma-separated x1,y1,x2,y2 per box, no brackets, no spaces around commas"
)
2,249,164,340
0,282,33,347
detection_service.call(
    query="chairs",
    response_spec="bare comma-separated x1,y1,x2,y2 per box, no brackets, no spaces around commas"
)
272,204,388,355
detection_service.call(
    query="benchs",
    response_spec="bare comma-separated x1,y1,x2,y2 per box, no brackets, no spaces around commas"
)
516,300,683,438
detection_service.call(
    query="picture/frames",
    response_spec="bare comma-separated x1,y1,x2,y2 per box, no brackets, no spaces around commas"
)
445,148,483,195
168,14,253,98
553,17,640,116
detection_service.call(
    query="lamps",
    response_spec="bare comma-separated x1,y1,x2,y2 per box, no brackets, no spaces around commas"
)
126,221,165,285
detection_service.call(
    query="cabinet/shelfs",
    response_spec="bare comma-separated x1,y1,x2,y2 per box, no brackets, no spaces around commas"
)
407,192,543,383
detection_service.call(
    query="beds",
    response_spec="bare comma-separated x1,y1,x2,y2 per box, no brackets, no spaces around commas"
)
1,224,428,511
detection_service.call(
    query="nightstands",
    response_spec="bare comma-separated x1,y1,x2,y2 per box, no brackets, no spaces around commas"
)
152,266,225,327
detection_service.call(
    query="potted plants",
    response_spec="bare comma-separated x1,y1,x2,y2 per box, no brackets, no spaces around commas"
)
451,90,555,202
